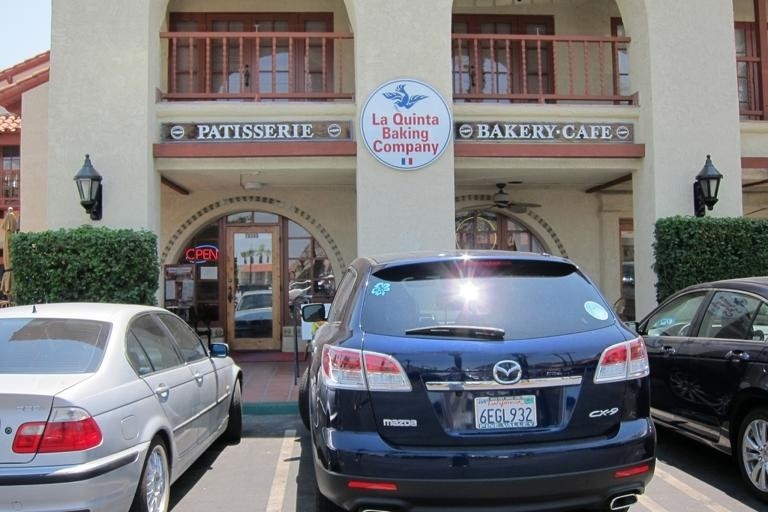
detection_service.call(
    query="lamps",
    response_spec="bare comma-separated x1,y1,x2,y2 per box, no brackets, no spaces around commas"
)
70,153,104,219
690,151,725,218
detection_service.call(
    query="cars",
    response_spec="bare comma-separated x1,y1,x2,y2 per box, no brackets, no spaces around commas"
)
235,274,335,336
0,302,245,512
234,289,273,338
621,274,768,502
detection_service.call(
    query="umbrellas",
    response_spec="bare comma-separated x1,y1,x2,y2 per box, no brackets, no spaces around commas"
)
0,207,20,306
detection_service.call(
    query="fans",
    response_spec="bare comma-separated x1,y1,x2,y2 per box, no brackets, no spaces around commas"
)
460,183,543,218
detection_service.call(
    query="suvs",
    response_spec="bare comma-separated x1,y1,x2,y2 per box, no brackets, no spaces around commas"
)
295,248,658,512
